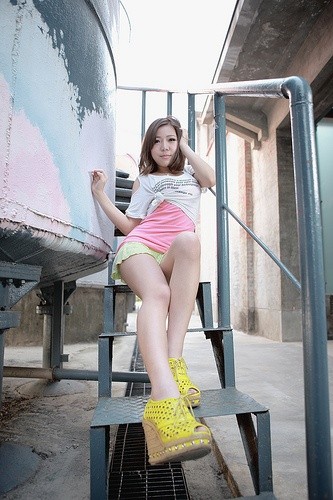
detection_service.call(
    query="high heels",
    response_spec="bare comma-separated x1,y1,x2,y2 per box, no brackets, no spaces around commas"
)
141,396,213,467
167,358,201,408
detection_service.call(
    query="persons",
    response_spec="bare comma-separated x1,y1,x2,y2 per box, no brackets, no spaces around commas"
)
90,115,214,466
